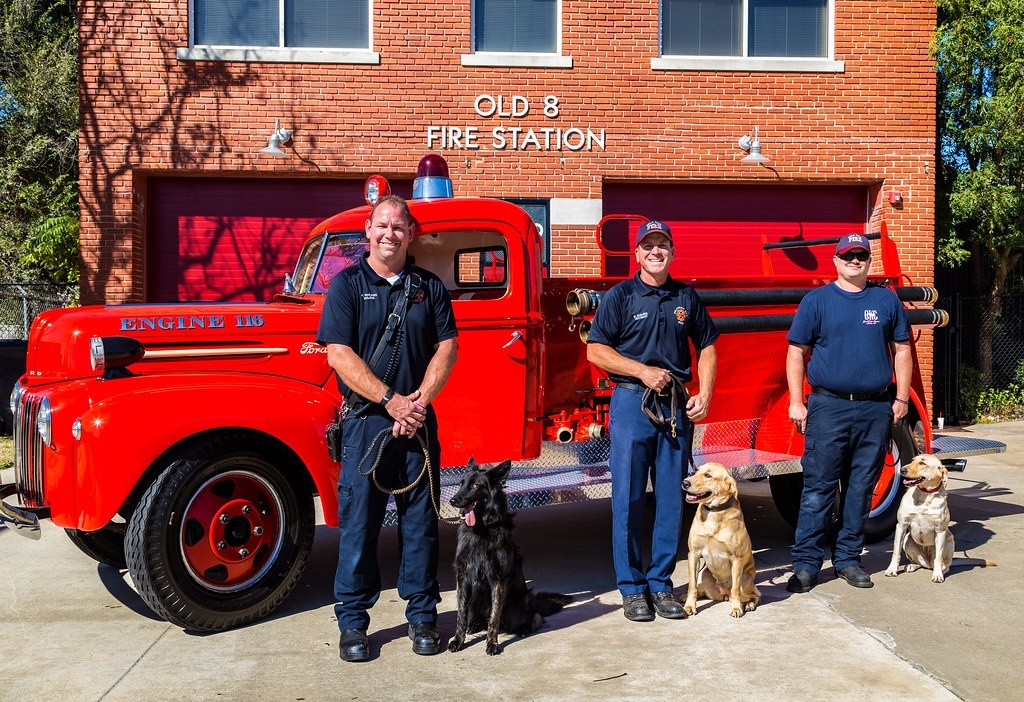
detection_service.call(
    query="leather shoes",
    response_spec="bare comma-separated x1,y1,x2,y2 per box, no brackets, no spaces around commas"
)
786,570,817,593
647,591,687,618
623,593,652,621
834,564,874,588
409,622,441,655
340,628,370,660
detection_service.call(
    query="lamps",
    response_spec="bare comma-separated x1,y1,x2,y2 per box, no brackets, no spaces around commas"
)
256,119,294,158
738,125,770,164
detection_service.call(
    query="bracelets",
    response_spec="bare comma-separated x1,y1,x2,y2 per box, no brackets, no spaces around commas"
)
380,388,395,407
895,398,909,404
413,401,426,410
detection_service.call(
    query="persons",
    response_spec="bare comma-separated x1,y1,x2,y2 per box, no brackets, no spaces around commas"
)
586,221,719,622
314,195,459,660
786,234,914,592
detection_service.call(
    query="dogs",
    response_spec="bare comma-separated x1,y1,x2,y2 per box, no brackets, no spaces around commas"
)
884,453,1000,584
682,463,763,618
447,454,574,655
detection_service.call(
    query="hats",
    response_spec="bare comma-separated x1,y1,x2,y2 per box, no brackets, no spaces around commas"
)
835,234,870,256
637,221,673,247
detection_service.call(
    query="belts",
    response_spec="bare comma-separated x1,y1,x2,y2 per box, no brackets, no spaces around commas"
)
617,381,682,397
811,385,888,402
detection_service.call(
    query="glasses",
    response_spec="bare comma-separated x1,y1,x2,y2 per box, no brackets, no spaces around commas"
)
836,250,869,261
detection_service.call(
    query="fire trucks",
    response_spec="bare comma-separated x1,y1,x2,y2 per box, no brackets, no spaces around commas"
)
0,153,1007,633
